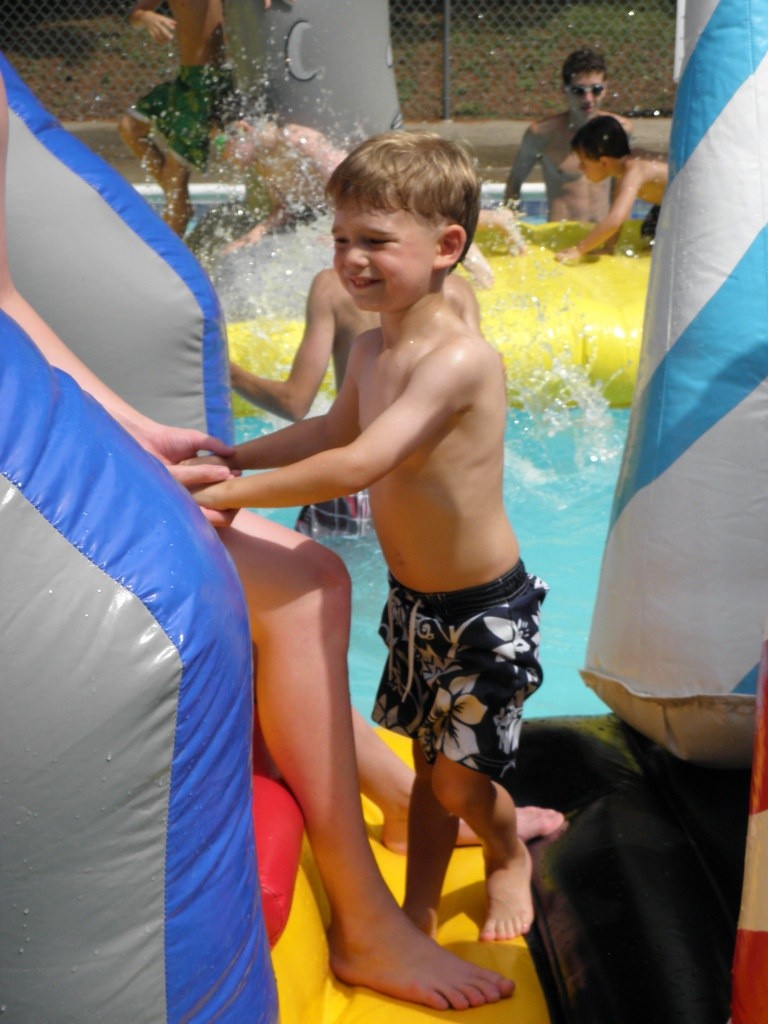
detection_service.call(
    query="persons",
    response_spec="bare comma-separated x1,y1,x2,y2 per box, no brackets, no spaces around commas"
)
506,47,634,224
119,0,271,237
178,130,546,942
226,268,480,541
0,68,565,1010
556,116,668,263
208,84,528,288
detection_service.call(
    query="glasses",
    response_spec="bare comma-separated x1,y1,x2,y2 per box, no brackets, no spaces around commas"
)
211,135,228,160
571,84,604,94
